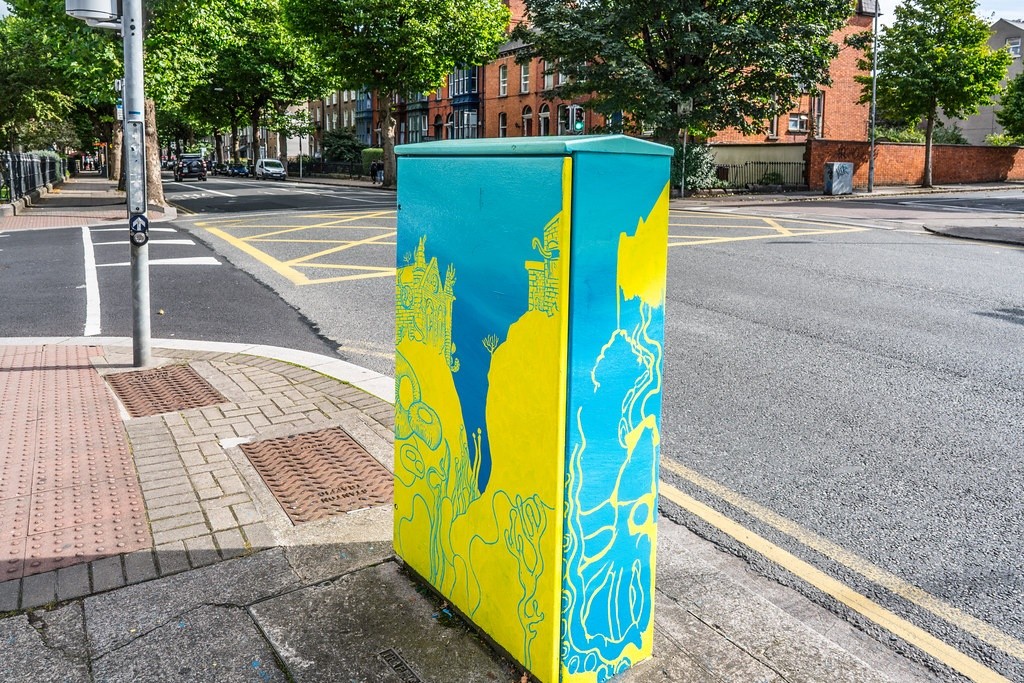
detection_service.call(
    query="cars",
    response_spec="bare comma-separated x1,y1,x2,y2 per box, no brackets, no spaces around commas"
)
228,163,250,178
210,164,232,177
162,160,177,171
207,160,217,171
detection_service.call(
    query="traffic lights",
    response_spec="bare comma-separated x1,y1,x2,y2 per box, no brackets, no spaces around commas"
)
572,105,585,132
563,106,571,131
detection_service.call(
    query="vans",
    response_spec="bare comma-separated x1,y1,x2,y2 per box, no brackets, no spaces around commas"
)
255,158,287,181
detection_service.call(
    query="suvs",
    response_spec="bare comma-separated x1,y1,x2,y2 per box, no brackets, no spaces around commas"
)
173,153,207,181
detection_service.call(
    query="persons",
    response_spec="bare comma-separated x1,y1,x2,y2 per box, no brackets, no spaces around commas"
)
370,157,384,184
84,160,100,170
